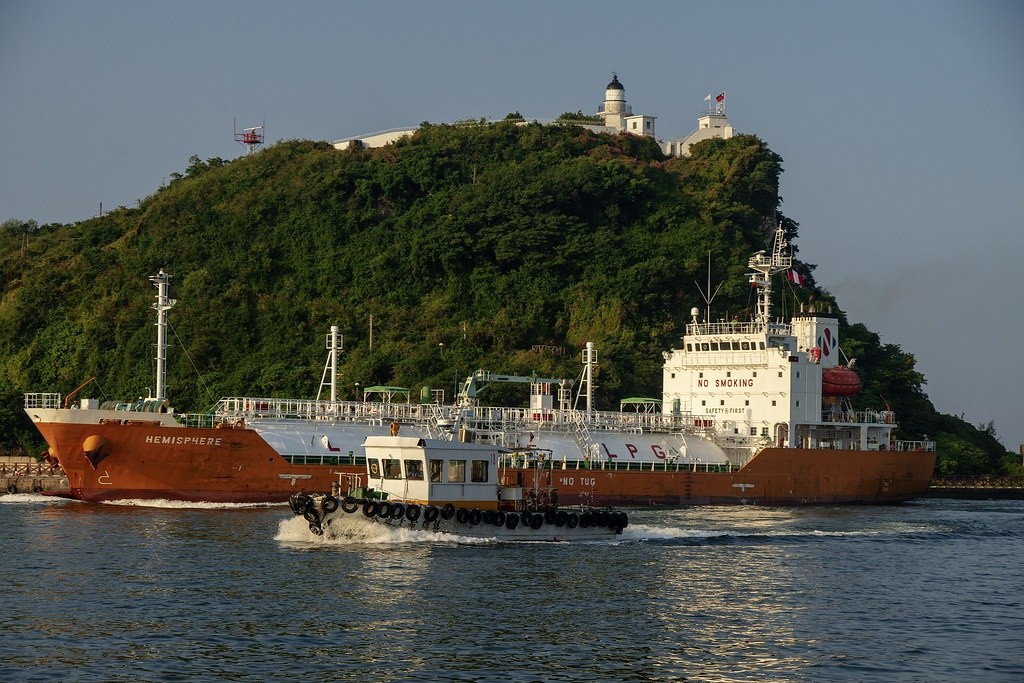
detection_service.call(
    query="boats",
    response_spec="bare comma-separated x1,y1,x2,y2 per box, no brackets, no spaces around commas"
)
287,395,629,541
23,219,938,508
821,369,862,396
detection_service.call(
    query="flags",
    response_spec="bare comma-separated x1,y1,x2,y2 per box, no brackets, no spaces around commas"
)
704,94,710,101
715,92,725,102
787,268,803,285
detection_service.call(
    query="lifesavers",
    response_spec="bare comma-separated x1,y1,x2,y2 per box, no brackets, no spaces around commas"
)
483,510,505,527
370,463,379,474
288,493,323,537
457,507,469,524
580,511,628,528
362,501,379,518
522,511,543,530
342,496,358,513
506,513,519,529
377,502,392,518
441,504,455,520
424,506,439,522
545,511,578,529
526,489,548,511
322,496,338,513
470,509,482,525
390,503,405,520
406,505,420,521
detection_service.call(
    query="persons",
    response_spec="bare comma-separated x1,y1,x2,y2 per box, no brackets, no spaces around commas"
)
923,434,931,452
407,463,421,478
71,400,79,409
